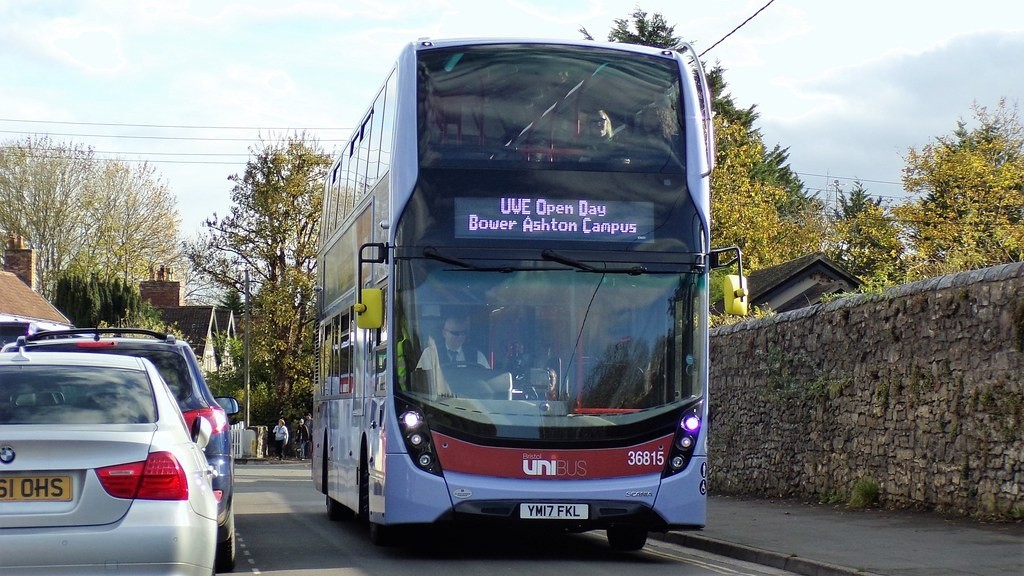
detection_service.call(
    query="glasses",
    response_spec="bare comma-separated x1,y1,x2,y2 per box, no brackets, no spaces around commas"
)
443,329,468,335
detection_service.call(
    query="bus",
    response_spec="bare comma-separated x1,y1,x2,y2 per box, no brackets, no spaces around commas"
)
311,35,747,551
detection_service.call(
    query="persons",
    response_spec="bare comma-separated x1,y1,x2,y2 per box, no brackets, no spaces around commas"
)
415,312,493,402
304,413,313,458
497,338,565,401
625,103,680,162
272,419,289,461
569,107,625,161
295,418,310,460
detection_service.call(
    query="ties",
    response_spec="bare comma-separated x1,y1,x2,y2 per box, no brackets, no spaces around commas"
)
448,349,459,369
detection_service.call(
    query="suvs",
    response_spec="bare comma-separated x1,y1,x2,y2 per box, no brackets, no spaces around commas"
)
2,321,242,570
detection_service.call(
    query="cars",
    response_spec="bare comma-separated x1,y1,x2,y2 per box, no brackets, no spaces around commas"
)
0,352,218,574
